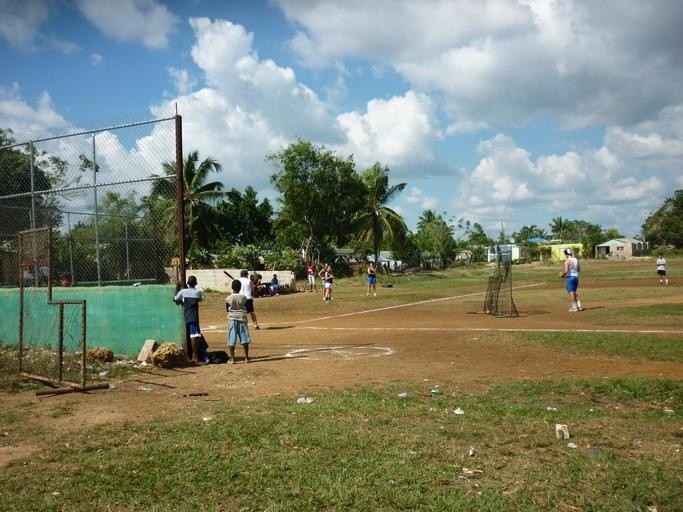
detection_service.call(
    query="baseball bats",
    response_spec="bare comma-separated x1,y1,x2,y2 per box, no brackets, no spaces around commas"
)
223,271,236,280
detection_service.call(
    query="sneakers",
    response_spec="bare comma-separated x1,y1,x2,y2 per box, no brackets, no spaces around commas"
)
322,296,334,303
365,292,377,297
567,306,583,313
658,281,668,285
255,325,261,330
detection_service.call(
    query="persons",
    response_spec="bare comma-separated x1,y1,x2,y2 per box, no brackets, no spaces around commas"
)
655,253,669,285
254,274,268,298
38,266,49,287
317,263,333,300
224,280,252,365
237,270,259,329
324,265,334,302
51,271,72,287
559,247,583,313
249,274,258,297
172,275,210,364
269,274,279,296
366,262,376,297
23,267,34,286
306,262,317,293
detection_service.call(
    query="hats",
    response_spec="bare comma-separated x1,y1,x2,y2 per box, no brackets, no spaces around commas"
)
240,270,249,276
563,249,572,256
